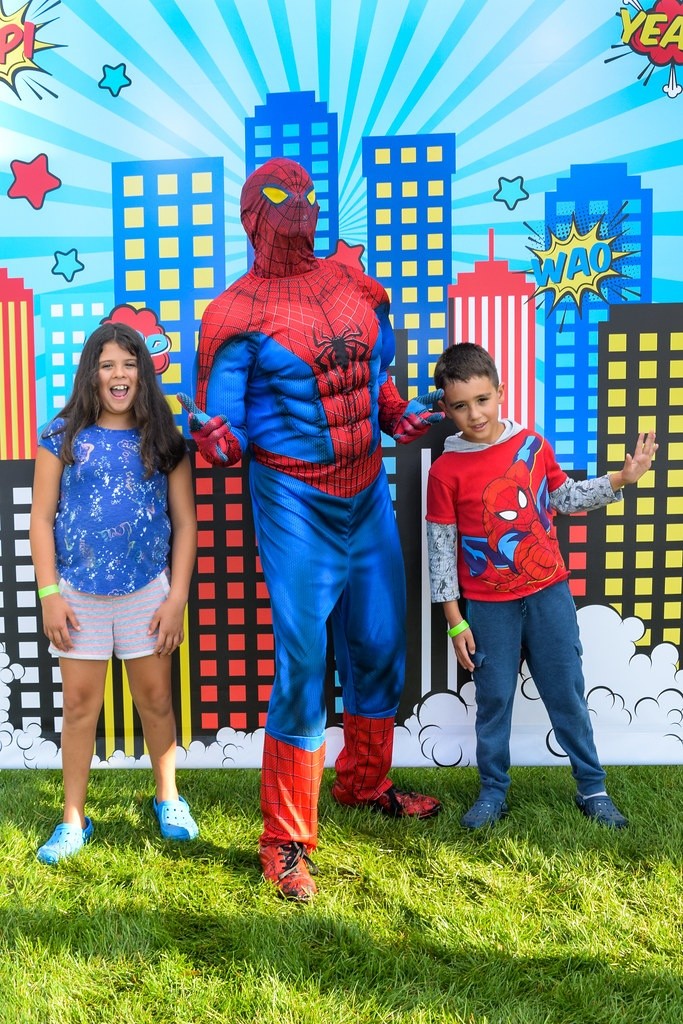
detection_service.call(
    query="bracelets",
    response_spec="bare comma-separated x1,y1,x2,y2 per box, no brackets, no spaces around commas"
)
447,620,470,637
38,584,60,598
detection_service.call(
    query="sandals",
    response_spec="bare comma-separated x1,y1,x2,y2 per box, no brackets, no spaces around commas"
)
154,794,198,843
461,797,506,830
575,792,629,829
38,815,93,864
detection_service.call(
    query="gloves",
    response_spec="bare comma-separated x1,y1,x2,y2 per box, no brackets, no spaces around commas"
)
175,392,233,466
391,388,448,446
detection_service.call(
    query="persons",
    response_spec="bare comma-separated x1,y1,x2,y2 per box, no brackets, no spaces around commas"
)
177,157,445,903
30,323,199,865
425,342,658,830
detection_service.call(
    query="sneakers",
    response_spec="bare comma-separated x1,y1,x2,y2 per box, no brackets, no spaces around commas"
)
344,785,442,820
259,843,319,904
